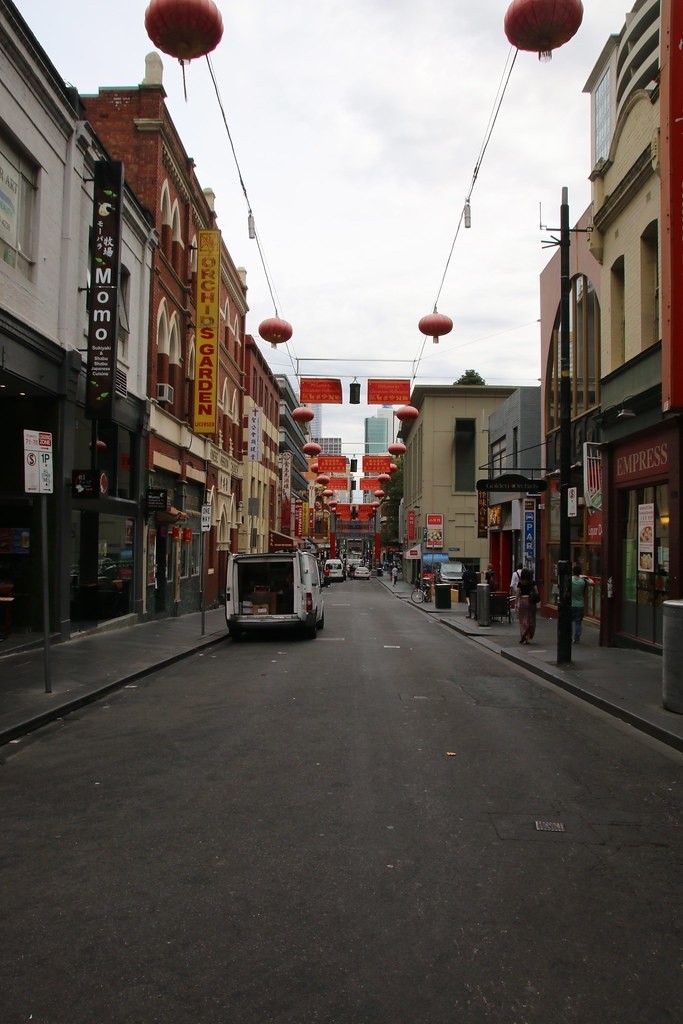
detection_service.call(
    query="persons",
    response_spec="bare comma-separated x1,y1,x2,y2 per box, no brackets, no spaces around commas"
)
462,563,477,607
510,562,540,645
383,559,400,587
325,567,331,577
349,564,355,580
571,565,595,643
484,562,497,592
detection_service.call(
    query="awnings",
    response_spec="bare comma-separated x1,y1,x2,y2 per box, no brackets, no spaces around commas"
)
268,530,297,553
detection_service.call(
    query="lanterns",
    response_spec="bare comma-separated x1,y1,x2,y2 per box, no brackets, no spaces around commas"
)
302,443,321,458
418,309,453,344
388,442,407,459
259,316,294,348
291,406,314,428
144,0,224,102
172,524,181,543
503,0,584,64
397,405,419,425
310,463,337,513
372,464,398,510
183,527,192,544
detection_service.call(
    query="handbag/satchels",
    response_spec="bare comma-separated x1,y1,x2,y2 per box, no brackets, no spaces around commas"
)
528,591,540,603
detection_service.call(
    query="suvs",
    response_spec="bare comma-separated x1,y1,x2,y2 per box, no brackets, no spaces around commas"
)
434,562,467,588
349,563,364,578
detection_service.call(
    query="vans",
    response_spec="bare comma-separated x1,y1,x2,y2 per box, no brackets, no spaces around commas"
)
346,553,363,566
324,558,344,582
225,551,325,640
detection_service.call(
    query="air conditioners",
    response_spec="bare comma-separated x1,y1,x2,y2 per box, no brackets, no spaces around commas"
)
157,383,175,404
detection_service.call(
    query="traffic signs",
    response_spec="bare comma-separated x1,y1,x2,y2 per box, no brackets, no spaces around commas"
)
201,506,211,531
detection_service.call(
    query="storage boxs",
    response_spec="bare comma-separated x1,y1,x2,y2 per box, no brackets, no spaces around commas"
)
240,583,277,615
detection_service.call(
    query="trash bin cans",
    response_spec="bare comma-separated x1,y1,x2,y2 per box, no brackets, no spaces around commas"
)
376,568,382,576
476,583,491,627
661,601,683,714
434,584,452,609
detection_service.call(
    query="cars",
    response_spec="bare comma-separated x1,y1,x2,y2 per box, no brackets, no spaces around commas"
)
354,567,369,580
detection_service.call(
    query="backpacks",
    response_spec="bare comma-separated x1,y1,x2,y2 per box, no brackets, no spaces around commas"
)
489,575,495,585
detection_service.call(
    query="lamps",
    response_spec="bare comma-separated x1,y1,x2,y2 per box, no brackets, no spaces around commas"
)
464,201,471,229
349,377,361,405
248,215,255,239
414,505,420,508
661,513,669,528
352,506,357,520
351,477,356,490
617,406,635,418
350,455,357,473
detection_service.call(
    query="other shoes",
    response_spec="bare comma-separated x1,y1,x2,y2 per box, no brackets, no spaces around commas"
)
518,639,524,643
574,632,580,644
526,641,530,644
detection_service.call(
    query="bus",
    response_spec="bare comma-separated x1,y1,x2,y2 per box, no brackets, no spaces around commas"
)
347,539,363,552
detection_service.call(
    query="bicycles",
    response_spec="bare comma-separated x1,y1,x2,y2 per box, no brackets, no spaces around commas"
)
411,579,431,604
